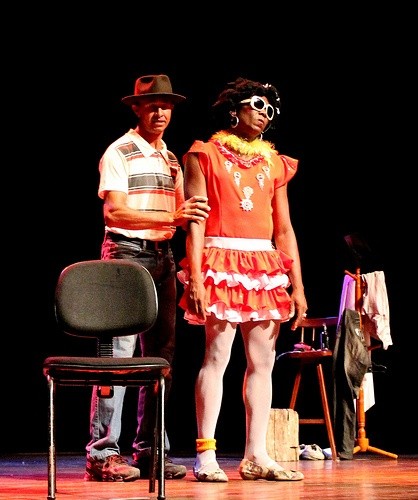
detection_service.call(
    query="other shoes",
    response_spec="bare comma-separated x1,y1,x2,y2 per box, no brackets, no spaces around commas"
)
323,448,332,459
239,458,303,481
299,444,325,460
192,462,228,482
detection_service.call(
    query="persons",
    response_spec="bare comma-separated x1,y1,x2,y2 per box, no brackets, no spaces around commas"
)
184,79,307,481
85,75,211,483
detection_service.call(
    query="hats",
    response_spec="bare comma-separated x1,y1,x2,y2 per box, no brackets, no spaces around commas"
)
122,75,186,105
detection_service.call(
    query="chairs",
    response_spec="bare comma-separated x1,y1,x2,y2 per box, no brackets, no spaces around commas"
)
44,259,170,500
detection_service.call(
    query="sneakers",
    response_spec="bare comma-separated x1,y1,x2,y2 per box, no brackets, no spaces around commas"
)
84,455,140,482
132,452,185,478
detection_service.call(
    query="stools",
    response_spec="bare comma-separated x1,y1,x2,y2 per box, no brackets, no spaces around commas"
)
280,316,339,460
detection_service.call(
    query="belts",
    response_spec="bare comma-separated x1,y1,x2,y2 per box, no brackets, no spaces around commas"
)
107,231,168,252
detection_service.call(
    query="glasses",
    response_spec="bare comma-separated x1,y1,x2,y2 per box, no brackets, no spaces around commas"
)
238,95,274,121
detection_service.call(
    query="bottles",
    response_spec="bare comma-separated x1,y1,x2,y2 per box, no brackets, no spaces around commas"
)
320,331,329,350
